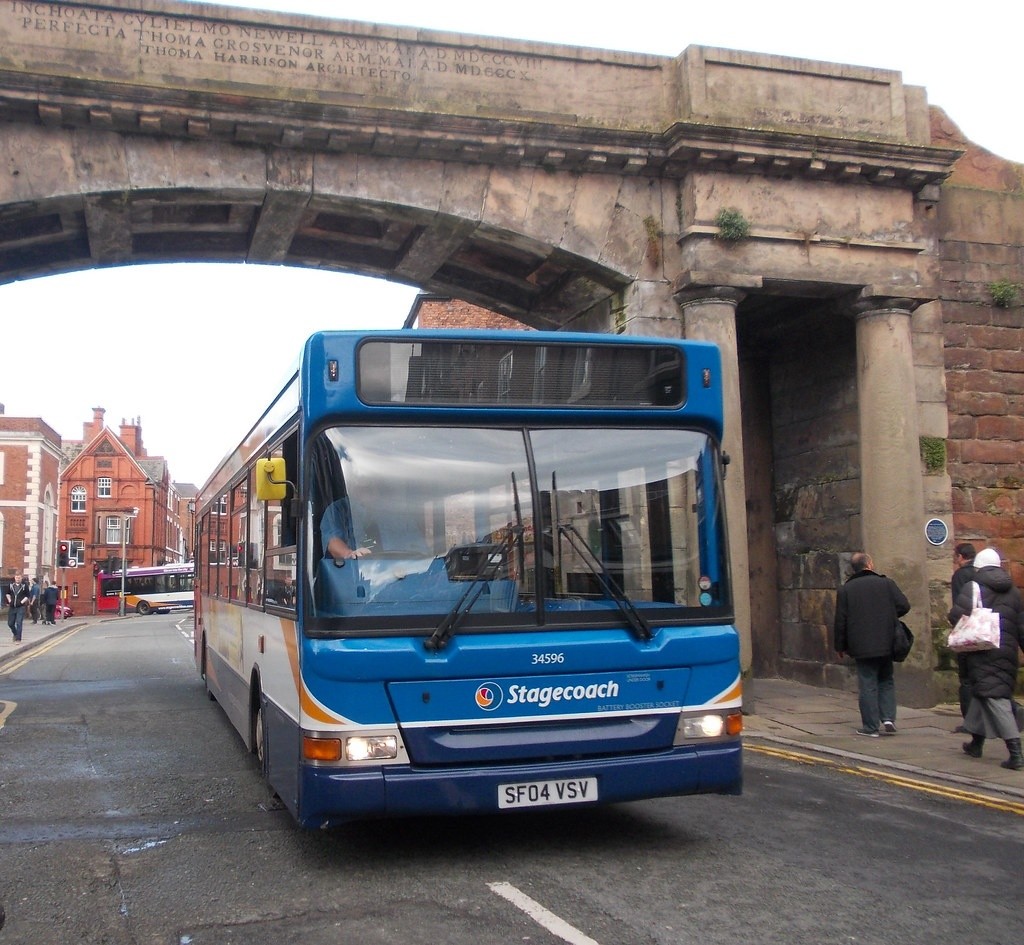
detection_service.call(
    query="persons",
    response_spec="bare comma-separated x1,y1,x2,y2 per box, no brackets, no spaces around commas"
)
5,571,30,644
39,580,59,626
319,464,426,559
947,544,1024,769
834,553,910,737
30,578,40,624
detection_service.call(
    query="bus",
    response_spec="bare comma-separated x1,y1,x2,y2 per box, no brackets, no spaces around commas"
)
99,564,196,616
191,326,743,823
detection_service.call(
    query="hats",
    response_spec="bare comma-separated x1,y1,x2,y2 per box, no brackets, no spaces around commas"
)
973,548,1001,568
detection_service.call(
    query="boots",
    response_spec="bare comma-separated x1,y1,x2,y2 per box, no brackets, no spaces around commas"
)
1000,737,1024,769
961,734,986,757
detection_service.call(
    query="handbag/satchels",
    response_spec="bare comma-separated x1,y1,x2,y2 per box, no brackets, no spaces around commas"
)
893,621,914,662
948,580,1000,652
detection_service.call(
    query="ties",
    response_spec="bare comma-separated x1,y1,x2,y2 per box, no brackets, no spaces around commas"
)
364,519,382,553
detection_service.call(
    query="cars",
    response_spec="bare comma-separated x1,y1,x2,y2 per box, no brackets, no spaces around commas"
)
39,593,74,619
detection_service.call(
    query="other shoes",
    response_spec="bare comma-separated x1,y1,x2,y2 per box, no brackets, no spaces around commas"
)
12,634,21,645
856,726,879,739
883,719,897,735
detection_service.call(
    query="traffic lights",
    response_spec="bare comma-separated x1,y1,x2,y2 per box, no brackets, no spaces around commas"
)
237,542,246,566
57,541,79,570
250,543,259,569
